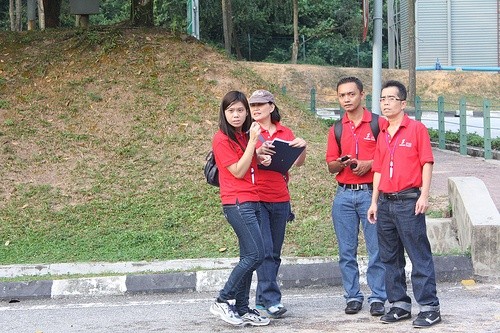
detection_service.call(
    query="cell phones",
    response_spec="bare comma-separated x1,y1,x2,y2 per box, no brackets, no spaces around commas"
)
342,155,348,162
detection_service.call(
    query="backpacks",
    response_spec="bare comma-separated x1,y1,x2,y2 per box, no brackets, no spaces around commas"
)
204,131,245,187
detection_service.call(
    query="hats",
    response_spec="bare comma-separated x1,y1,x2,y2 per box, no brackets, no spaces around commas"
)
249,89,275,104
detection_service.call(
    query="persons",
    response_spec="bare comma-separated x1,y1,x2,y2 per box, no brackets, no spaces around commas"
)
209,91,271,327
326,77,390,316
367,80,441,328
246,88,307,316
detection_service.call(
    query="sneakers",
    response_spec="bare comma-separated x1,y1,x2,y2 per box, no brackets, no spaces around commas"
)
240,305,271,326
255,301,287,319
345,301,362,314
370,302,385,315
380,306,412,324
412,310,441,328
209,294,245,327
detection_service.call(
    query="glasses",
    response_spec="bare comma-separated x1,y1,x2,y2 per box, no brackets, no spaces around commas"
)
379,96,402,102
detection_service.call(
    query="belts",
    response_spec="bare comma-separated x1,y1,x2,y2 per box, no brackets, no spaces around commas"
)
338,182,372,191
382,187,419,201
